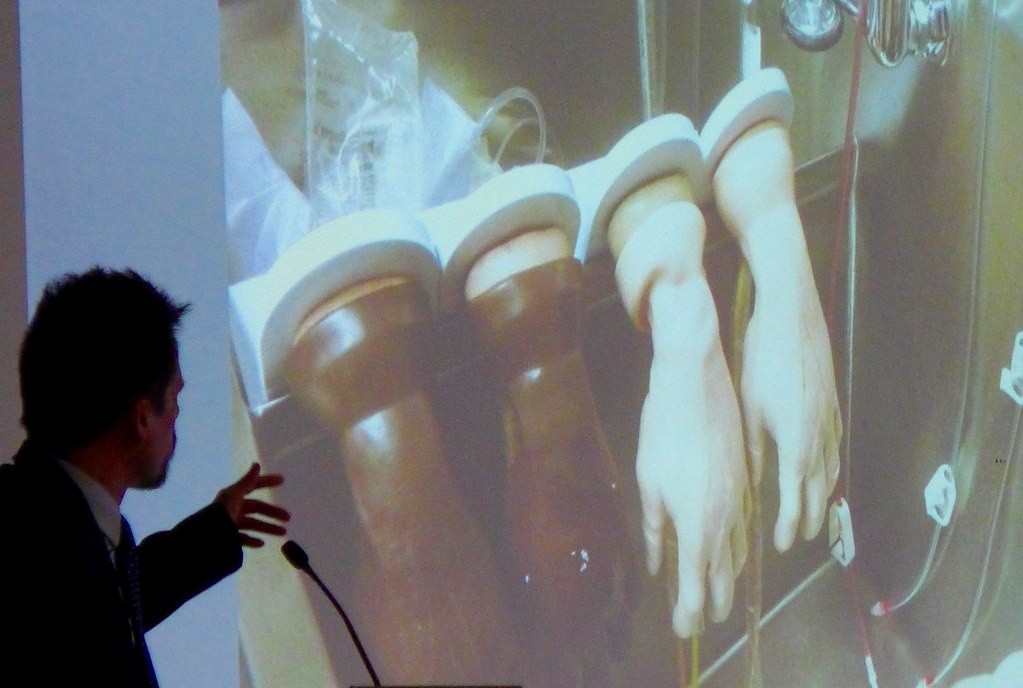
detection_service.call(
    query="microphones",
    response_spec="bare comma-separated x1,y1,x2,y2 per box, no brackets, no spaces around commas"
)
281,540,382,688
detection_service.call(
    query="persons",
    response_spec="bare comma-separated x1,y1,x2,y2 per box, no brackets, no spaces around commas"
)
287,117,843,688
0,265,291,688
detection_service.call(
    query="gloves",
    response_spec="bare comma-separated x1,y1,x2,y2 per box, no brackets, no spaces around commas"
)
463,255,634,688
284,283,523,688
713,121,843,553
615,201,753,641
